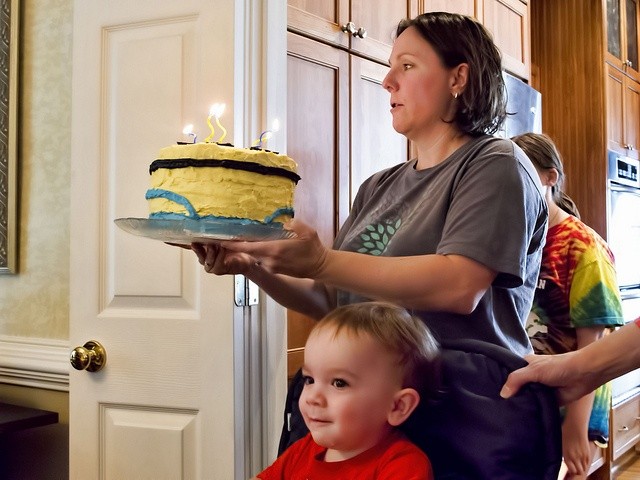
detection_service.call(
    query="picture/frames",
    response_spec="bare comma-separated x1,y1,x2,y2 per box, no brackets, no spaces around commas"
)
0,0,25,275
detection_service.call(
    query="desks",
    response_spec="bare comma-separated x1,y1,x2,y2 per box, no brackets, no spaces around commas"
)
0,402,61,434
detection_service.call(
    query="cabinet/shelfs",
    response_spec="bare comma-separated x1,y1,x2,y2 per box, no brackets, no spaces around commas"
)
288,1,408,66
419,0,532,83
286,28,407,382
531,1,640,480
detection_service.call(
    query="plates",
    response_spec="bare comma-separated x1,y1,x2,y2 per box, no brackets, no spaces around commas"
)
113,217,300,242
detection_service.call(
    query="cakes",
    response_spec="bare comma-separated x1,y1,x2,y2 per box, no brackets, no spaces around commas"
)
144,138,302,242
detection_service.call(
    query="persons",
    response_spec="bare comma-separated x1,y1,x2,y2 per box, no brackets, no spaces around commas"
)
499,315,640,408
165,11,548,355
509,133,625,480
253,300,444,480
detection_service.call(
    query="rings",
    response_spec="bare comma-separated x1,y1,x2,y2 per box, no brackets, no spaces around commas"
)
205,261,214,267
255,262,261,266
